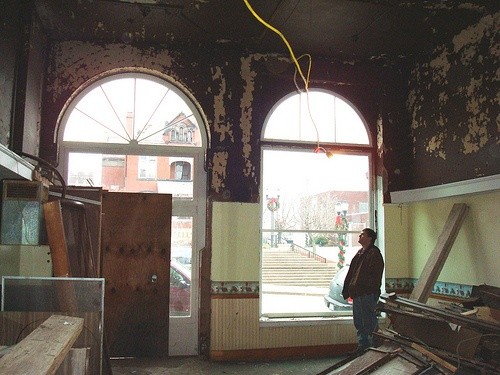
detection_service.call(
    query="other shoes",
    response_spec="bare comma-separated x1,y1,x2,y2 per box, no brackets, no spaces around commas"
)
348,347,360,355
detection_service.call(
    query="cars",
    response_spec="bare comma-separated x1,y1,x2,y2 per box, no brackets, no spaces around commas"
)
169,257,192,317
324,262,354,312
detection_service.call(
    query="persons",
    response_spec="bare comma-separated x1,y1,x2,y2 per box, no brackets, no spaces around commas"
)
341,228,385,353
